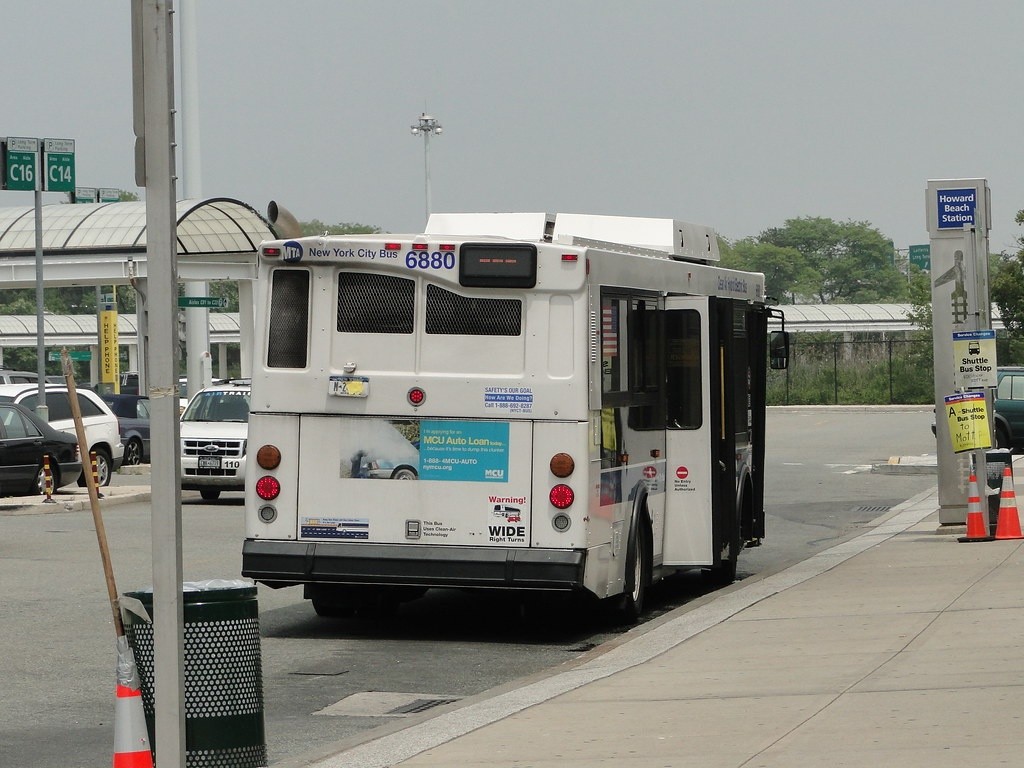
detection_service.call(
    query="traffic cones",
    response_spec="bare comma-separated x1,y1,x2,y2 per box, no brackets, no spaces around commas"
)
956,472,993,543
109,632,158,765
991,466,1023,542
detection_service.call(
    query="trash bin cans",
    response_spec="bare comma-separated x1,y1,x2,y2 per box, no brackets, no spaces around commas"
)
971,452,1014,536
120,579,269,767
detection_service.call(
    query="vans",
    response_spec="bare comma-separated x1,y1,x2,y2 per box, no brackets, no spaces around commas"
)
168,381,249,504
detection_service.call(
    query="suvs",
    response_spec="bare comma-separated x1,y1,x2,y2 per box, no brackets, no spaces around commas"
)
931,365,1022,457
0,379,126,490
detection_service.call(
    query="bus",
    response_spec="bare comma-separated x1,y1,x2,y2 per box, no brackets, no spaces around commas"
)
239,210,794,630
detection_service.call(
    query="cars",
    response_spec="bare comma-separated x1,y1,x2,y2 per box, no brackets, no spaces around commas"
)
101,394,150,466
1,394,85,497
